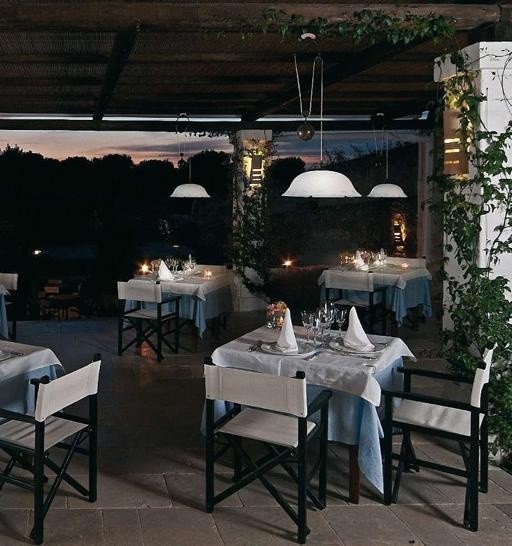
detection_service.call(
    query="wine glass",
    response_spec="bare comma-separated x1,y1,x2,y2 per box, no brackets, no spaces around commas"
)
377,248,387,267
301,301,347,352
165,256,196,277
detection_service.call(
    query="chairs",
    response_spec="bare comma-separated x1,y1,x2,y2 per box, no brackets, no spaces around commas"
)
117,281,179,363
324,270,386,336
0,353,101,545
204,357,333,544
380,339,495,532
1,273,20,337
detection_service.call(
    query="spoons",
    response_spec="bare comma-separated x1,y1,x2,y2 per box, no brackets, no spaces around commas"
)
248,339,263,352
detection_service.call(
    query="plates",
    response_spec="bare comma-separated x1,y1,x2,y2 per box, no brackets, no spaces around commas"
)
328,335,392,354
260,338,326,358
0,352,11,360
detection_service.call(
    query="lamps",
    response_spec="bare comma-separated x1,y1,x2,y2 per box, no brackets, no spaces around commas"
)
281,53,363,198
363,125,408,198
169,113,211,198
249,155,264,184
442,110,469,175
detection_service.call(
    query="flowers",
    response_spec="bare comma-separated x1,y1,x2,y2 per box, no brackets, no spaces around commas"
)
265,299,285,329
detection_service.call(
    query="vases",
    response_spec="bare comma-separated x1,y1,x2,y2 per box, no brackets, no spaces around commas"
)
272,327,281,340
